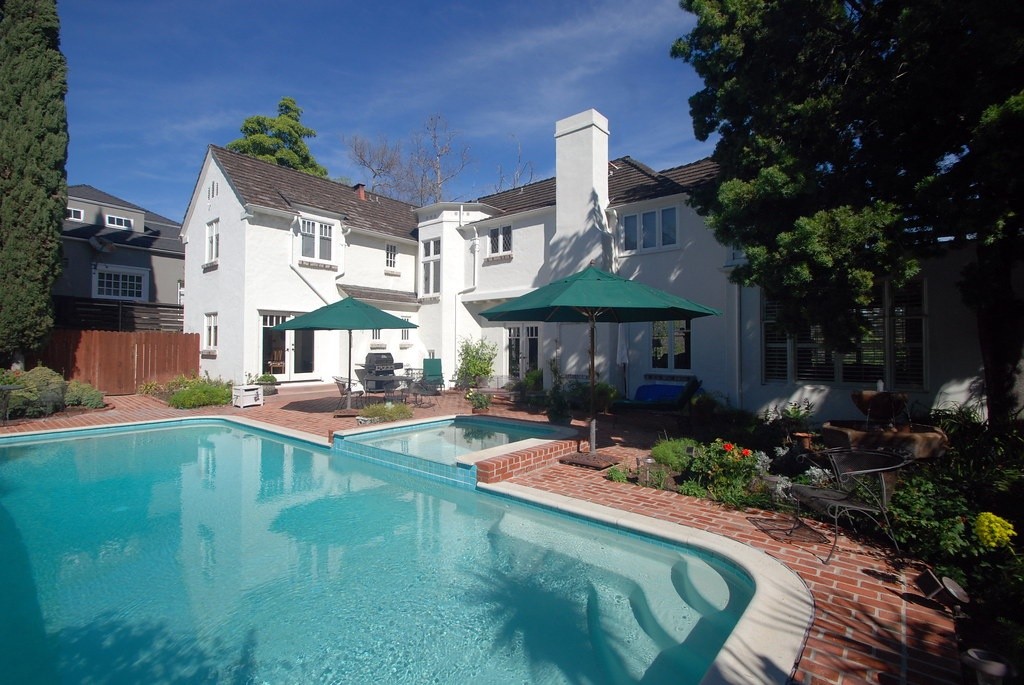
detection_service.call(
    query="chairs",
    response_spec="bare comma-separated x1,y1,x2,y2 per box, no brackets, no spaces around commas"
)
788,445,915,565
268,350,285,374
383,368,443,409
613,375,703,432
333,376,364,410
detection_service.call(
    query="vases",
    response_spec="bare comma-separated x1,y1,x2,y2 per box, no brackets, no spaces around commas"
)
472,408,489,413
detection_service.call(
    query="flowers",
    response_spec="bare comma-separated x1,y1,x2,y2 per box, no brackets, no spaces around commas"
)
464,388,496,410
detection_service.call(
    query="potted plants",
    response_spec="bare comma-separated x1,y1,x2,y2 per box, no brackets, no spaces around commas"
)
256,376,280,395
502,381,532,402
545,392,573,425
356,400,415,427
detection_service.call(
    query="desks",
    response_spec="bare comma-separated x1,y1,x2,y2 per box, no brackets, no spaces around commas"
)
0,385,26,427
364,375,415,404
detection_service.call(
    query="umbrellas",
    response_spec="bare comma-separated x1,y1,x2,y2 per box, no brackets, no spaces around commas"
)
271,298,420,410
477,262,724,453
